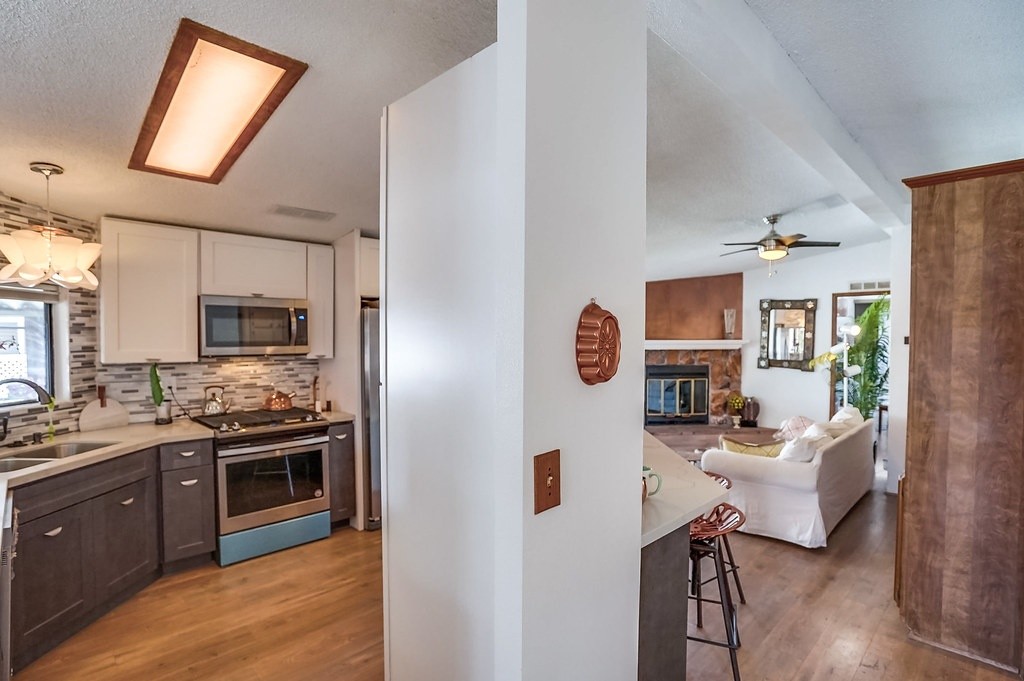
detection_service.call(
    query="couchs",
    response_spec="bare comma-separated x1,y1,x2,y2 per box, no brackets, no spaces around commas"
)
701,415,877,549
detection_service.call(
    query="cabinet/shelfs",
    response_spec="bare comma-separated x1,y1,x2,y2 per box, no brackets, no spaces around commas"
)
6,445,160,675
201,229,307,299
329,422,355,523
307,243,335,359
160,439,218,575
98,215,200,365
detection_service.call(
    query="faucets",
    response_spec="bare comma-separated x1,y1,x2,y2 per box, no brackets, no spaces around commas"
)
0,378,54,405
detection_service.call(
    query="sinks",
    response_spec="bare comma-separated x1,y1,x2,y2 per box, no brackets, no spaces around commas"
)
0,442,123,459
0,460,54,473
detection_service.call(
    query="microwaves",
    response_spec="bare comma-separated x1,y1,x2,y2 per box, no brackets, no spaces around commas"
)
199,294,311,359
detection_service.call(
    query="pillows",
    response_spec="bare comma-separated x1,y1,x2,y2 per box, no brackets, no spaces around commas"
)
719,437,784,459
804,423,848,438
777,435,825,462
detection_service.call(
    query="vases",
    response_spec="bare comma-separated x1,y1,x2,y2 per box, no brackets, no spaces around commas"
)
732,415,742,429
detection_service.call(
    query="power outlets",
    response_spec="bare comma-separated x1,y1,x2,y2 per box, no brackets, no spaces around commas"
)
161,375,177,394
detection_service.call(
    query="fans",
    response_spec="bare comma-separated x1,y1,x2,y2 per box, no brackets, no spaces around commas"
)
721,215,841,257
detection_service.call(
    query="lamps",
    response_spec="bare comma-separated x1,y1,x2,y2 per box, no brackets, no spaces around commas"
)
758,240,789,261
831,325,861,405
0,161,103,291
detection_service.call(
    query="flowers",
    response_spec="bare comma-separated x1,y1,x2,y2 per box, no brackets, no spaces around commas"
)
729,392,744,415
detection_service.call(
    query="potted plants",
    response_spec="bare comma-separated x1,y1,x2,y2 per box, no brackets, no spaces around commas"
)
809,291,892,464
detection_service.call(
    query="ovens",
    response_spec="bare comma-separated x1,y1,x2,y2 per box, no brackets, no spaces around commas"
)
215,421,331,567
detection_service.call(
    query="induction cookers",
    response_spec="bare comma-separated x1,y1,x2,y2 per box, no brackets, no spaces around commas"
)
195,406,319,429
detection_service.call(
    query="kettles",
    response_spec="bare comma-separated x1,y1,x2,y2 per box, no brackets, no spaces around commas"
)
262,390,296,411
201,386,232,416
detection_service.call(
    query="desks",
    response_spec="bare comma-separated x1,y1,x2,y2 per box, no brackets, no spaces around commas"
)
637,429,731,681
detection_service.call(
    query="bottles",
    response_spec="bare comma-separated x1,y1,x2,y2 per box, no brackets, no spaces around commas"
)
326,400,332,417
315,400,323,419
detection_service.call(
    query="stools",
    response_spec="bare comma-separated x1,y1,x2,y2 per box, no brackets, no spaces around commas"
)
686,471,747,681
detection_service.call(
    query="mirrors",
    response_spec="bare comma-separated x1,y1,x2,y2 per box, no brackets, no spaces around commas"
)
756,298,817,372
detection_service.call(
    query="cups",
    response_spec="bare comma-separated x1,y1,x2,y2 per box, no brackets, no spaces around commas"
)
155,399,173,425
642,466,661,504
